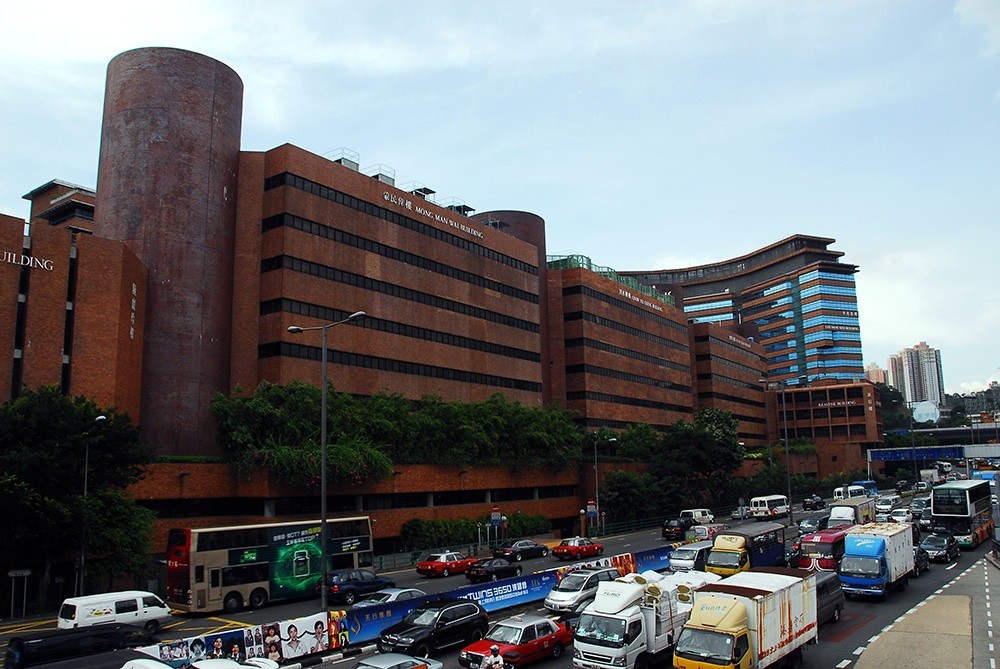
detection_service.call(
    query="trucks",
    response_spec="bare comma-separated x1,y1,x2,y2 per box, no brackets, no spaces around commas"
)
672,565,821,669
571,568,722,669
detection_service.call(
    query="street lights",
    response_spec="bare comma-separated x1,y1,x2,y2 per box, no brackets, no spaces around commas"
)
77,414,109,596
285,310,372,610
591,430,620,535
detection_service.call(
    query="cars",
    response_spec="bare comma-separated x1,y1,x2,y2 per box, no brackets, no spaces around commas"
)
465,556,525,584
493,539,550,561
655,444,1000,629
416,551,481,577
552,535,605,560
459,612,577,668
348,653,446,668
315,565,399,605
183,657,280,669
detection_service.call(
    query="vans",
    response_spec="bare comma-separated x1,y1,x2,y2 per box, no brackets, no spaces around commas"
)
5,621,161,665
56,589,174,634
543,566,623,618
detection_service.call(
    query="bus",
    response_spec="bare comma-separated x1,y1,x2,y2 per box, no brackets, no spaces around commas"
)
163,514,376,614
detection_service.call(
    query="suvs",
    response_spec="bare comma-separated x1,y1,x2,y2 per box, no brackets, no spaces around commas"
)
377,597,492,661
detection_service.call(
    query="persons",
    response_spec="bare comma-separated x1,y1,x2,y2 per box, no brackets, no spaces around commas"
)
309,621,329,654
558,558,610,583
483,644,504,669
206,636,227,659
263,622,281,644
283,624,308,658
226,641,245,664
245,624,265,661
328,610,350,649
160,640,190,669
268,643,280,662
190,638,206,662
